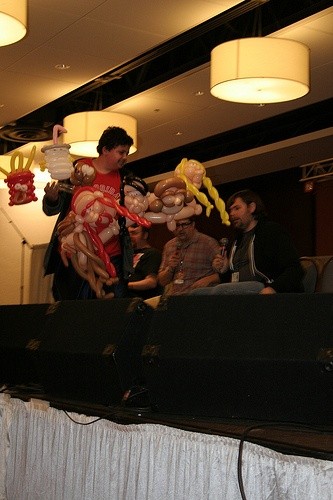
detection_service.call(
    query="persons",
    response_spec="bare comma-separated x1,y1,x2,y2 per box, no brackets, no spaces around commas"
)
43,126,149,301
157,213,220,296
188,191,304,296
103,220,164,299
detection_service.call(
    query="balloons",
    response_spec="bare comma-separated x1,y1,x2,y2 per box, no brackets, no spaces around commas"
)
0,124,74,206
56,157,231,299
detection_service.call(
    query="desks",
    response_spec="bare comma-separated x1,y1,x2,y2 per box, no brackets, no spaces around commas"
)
0,292,333,429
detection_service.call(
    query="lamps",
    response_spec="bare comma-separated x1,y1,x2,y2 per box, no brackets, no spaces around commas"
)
210,37,310,105
63,111,137,158
1,0,27,47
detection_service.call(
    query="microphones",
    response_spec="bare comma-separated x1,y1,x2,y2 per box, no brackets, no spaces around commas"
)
219,237,229,257
175,240,181,272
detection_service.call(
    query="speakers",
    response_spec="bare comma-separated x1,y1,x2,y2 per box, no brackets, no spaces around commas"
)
146,294,333,426
29,297,154,405
0,303,52,385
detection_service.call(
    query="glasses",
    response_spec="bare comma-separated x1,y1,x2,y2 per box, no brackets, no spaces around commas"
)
176,222,192,228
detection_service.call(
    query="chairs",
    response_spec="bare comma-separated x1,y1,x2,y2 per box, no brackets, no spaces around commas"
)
298,257,333,294
